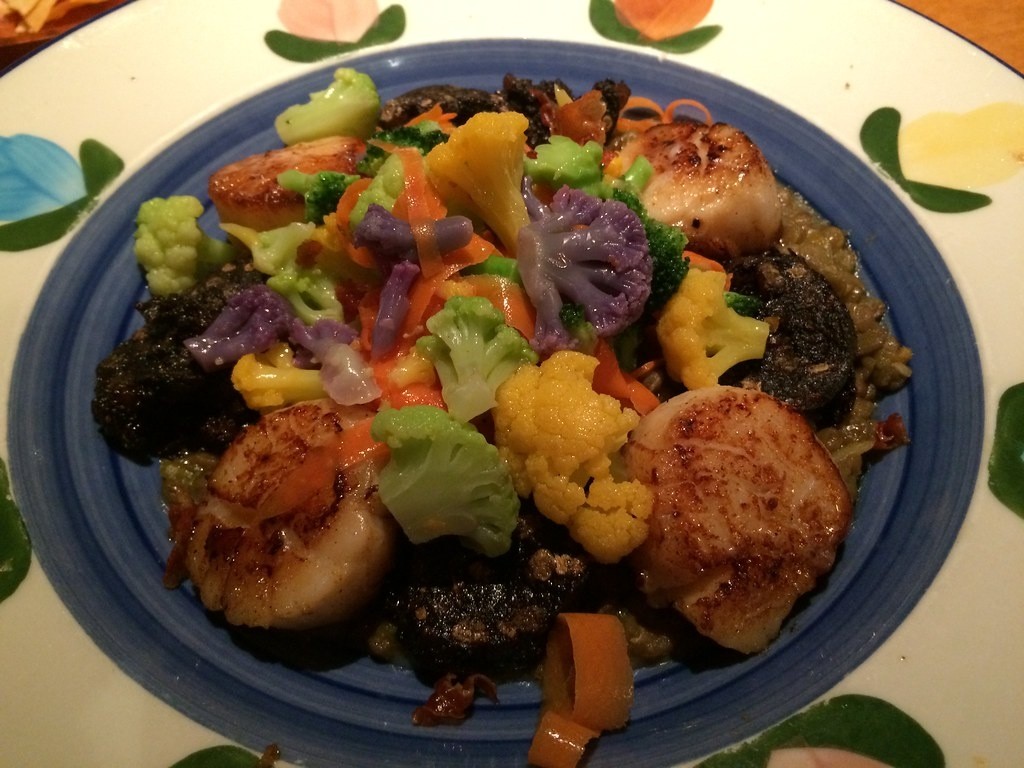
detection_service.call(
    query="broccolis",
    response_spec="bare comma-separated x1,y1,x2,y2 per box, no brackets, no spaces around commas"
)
131,67,772,561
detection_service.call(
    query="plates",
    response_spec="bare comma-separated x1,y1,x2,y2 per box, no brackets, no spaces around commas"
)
3,31,987,768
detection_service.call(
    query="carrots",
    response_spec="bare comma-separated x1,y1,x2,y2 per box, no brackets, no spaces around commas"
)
258,97,728,768
